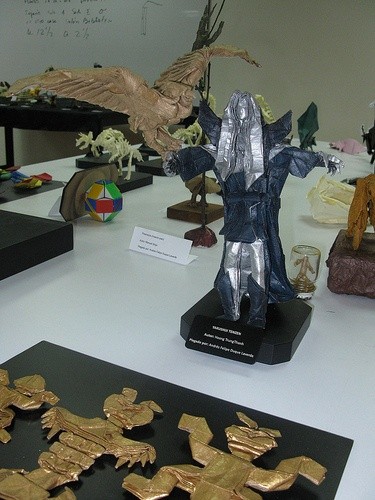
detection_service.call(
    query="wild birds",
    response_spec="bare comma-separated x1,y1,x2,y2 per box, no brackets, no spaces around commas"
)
4,44,263,162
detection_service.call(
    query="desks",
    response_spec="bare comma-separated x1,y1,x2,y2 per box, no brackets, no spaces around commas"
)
0,137,375,499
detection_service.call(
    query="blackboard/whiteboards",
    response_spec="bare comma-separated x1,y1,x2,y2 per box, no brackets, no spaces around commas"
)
0,0,207,101
